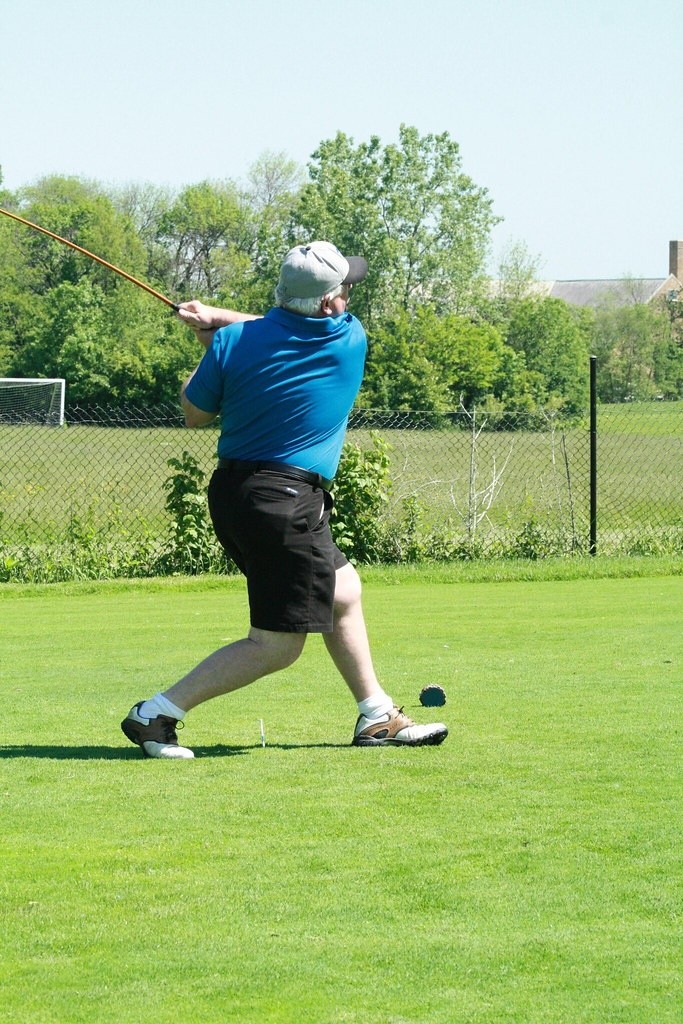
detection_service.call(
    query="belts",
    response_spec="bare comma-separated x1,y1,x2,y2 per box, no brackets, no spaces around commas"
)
218,458,333,492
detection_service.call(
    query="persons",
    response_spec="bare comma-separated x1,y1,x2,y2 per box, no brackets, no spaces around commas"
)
121,242,448,758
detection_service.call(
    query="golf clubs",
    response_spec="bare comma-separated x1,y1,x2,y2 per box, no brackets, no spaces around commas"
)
0,207,184,312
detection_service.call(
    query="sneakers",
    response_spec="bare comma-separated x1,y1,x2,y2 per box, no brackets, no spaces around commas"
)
122,700,194,759
354,704,448,747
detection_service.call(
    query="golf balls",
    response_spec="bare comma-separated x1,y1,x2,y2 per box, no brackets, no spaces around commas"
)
418,682,446,707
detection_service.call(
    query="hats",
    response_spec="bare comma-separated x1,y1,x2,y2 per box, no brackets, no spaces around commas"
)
280,241,368,299
